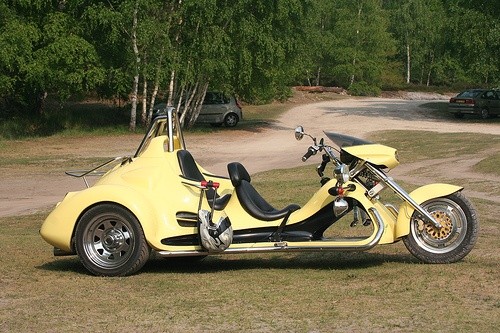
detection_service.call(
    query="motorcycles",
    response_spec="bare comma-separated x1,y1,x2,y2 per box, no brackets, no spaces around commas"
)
39,108,478,277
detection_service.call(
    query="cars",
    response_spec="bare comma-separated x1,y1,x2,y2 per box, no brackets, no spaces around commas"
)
147,89,243,128
447,89,500,119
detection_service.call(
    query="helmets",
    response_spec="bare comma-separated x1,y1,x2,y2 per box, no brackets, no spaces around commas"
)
199,209,233,254
